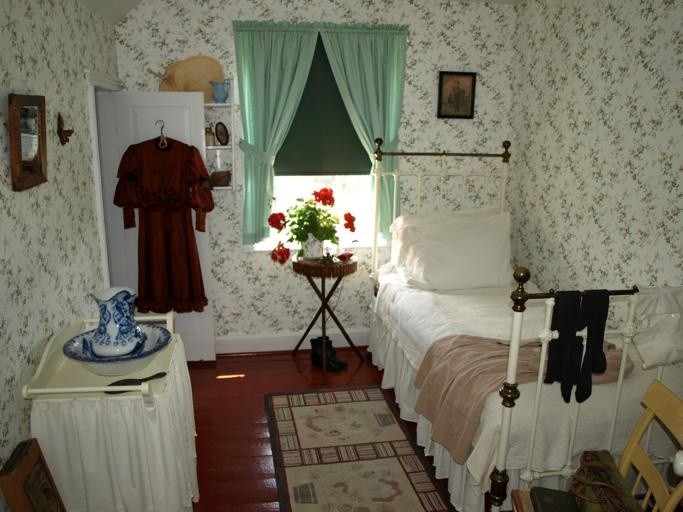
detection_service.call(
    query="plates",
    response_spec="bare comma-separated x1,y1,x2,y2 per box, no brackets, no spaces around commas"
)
216,121,229,145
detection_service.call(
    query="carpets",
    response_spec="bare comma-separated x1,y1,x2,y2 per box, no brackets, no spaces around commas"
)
266,385,452,512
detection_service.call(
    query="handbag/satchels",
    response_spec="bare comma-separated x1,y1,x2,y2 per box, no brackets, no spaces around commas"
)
571,449,647,512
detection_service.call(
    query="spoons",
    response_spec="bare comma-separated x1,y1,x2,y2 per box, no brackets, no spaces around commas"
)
104,371,167,395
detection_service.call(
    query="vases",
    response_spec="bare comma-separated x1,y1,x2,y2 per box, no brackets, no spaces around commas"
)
297,232,324,260
89,286,143,359
206,127,215,146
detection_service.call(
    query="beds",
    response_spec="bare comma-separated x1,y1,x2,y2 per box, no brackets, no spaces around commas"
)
359,139,683,512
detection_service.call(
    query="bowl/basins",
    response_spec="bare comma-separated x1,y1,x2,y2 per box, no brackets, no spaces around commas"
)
62,321,173,376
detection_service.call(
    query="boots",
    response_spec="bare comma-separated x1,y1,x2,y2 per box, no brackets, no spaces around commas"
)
317,337,348,369
310,339,338,372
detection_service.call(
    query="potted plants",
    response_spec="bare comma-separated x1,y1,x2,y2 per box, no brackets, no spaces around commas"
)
208,154,232,186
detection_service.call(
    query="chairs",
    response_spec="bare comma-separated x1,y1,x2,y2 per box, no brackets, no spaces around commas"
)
511,381,682,512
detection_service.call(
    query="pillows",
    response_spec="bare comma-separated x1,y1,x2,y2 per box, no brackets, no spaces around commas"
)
402,213,509,287
392,207,503,279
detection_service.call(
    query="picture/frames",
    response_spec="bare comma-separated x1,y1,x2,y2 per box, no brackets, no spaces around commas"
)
8,94,48,192
436,70,477,120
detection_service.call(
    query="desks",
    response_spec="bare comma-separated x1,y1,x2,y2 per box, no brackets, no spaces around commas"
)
23,310,197,511
290,261,366,383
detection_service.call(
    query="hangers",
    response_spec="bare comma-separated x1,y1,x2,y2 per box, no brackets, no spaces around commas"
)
152,118,175,149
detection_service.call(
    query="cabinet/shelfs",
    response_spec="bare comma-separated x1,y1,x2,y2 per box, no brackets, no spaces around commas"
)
205,102,236,191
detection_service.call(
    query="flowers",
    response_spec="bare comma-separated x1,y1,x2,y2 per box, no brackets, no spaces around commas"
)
264,187,355,265
